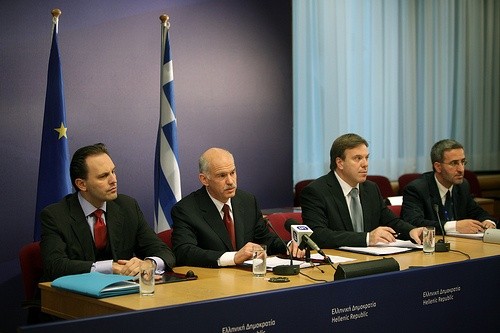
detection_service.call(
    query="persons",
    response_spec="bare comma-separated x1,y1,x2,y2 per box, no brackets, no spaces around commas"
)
399,139,500,237
171,147,309,269
37,142,176,282
299,133,435,251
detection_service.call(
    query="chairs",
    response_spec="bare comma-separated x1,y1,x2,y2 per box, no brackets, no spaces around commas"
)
293,170,481,219
263,212,304,242
21,242,45,306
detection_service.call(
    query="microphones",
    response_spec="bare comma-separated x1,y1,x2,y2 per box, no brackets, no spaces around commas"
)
433,204,450,252
264,217,300,275
284,218,325,256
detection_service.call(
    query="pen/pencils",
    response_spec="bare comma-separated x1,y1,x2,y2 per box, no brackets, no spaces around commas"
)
392,233,400,235
481,226,487,229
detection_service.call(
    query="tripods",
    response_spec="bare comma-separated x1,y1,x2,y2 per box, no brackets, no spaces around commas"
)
299,246,325,273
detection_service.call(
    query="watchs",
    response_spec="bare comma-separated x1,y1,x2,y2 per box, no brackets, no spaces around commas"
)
146,258,157,273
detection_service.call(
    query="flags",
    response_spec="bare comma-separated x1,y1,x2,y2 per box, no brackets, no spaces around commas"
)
33,18,73,242
152,24,183,250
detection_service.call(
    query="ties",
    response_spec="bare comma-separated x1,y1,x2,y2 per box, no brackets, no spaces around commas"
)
89,209,108,261
444,189,454,221
222,203,236,251
349,187,364,232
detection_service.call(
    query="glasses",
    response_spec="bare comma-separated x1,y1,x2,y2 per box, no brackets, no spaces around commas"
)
438,160,467,166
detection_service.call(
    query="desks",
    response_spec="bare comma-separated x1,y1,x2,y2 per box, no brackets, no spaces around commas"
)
38,235,500,320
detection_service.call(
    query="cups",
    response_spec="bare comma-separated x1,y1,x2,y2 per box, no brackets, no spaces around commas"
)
252,245,267,278
138,260,157,296
423,226,436,255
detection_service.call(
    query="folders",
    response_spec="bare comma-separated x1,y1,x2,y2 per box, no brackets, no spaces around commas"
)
50,272,141,299
